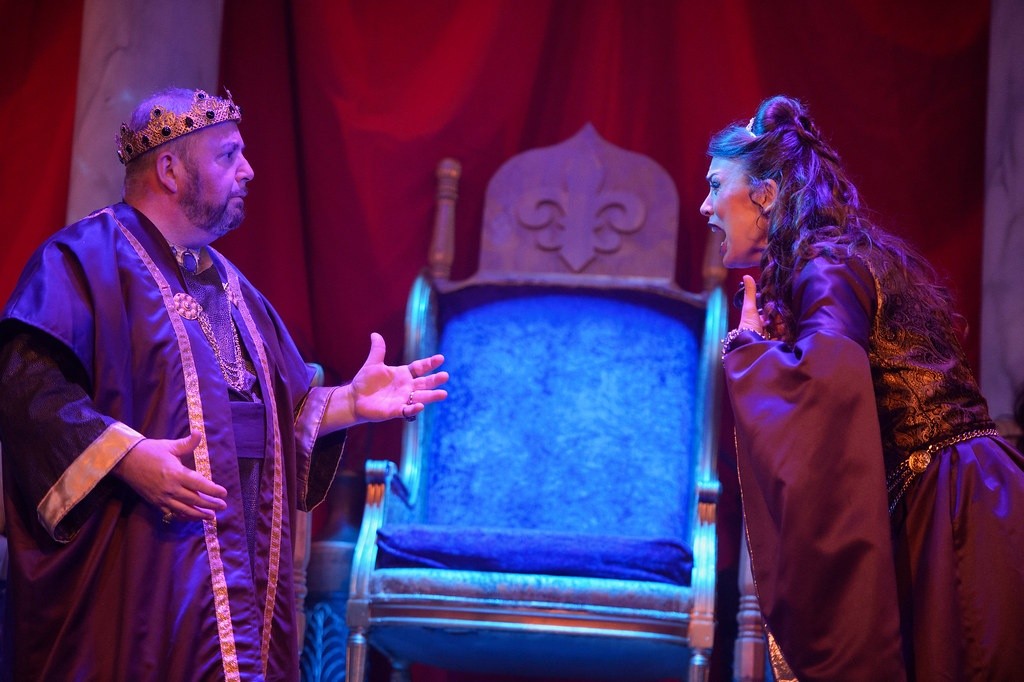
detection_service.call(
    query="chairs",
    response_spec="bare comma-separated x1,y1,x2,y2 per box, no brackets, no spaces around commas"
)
342,123,729,682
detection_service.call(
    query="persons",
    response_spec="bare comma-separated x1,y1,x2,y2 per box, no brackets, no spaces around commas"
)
0,84,457,682
697,92,1024,682
